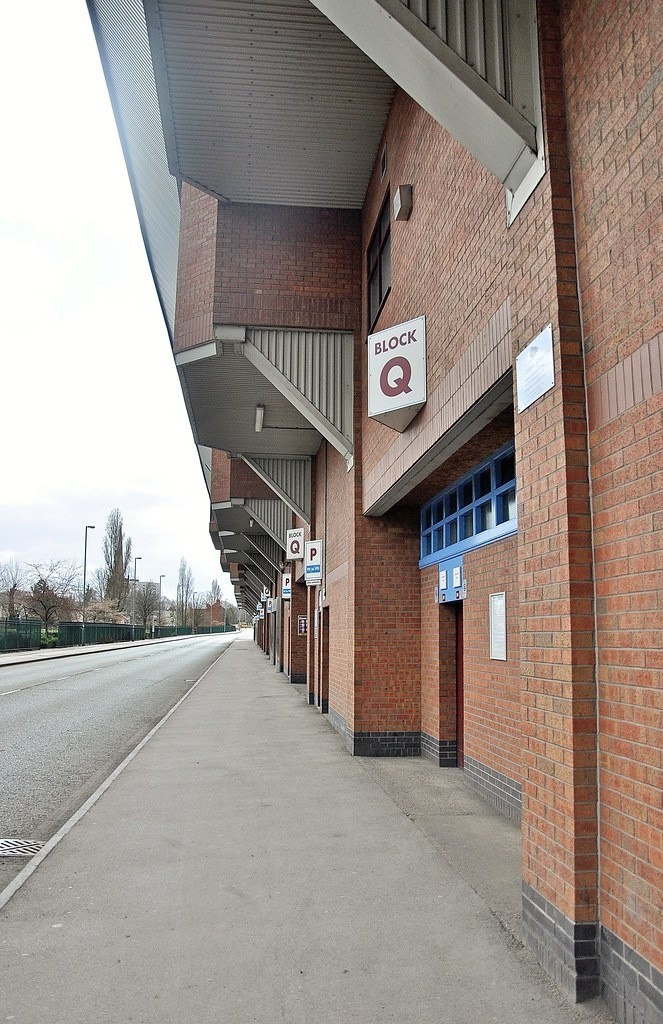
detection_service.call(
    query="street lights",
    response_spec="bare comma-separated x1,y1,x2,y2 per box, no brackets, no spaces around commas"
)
191,591,198,635
158,574,165,637
131,557,142,641
81,524,96,645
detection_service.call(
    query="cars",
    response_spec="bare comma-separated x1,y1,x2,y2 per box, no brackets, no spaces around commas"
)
239,622,248,628
